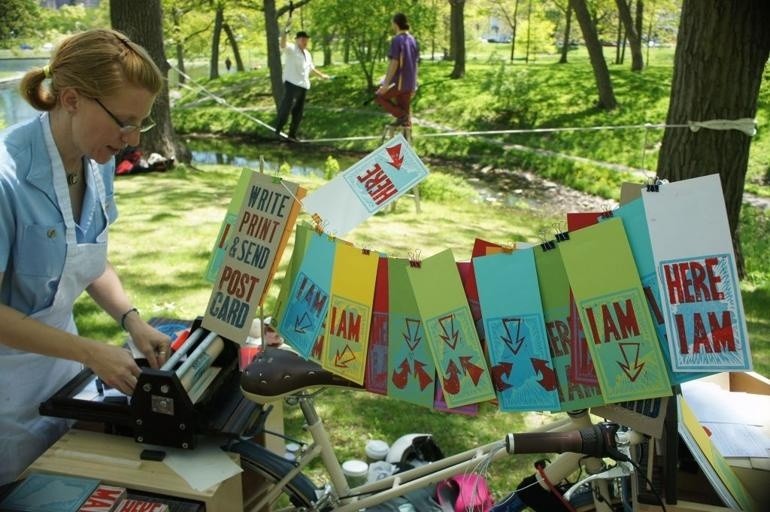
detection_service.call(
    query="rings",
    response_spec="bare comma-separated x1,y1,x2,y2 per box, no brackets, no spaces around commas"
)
159,352,166,354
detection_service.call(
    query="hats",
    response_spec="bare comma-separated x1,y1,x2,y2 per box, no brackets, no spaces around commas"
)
294,31,310,40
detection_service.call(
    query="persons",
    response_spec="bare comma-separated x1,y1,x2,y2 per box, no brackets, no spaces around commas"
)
275,31,330,143
0,29,171,485
375,13,419,127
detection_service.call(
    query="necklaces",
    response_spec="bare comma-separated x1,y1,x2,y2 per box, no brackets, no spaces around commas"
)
67,174,80,185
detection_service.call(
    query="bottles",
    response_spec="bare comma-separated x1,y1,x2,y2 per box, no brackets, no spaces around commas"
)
340,440,390,488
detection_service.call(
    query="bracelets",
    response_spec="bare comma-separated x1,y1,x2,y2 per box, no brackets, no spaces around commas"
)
121,308,138,329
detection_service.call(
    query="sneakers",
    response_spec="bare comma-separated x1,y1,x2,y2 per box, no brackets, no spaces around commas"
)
390,114,410,127
274,127,281,136
287,133,300,142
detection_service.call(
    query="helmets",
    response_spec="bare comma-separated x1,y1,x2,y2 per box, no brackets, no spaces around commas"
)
435,473,492,512
385,434,444,472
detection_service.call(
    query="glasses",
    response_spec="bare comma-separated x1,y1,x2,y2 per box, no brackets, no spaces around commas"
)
94,96,155,135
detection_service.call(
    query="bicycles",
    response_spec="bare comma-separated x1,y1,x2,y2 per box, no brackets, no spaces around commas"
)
221,347,666,512
